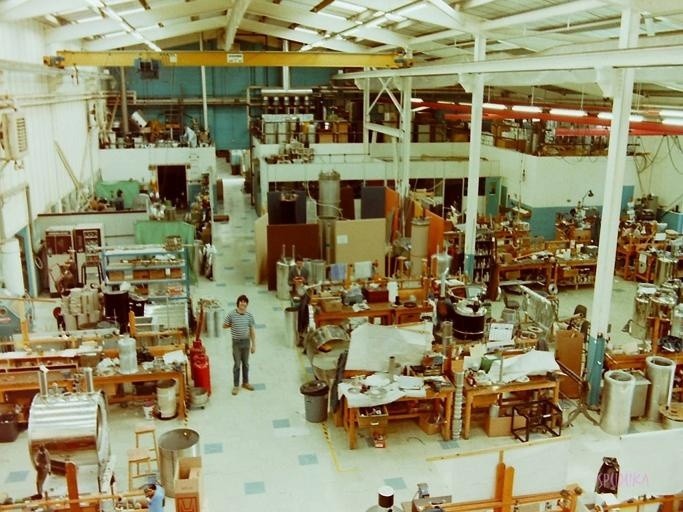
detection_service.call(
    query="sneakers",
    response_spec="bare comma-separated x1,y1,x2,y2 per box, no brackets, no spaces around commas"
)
242,383,254,391
232,386,239,395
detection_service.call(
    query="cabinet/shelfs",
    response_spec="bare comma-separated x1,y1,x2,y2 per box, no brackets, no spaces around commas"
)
41,218,194,337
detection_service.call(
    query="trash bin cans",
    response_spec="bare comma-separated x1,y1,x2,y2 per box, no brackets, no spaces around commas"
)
0,414,19,442
286,307,300,347
301,381,328,423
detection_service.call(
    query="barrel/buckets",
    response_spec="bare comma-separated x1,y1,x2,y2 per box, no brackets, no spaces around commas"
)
155,378,177,421
453,308,487,342
159,429,201,499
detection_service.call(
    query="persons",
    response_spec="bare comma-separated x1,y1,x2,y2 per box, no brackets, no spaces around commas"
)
112,188,126,210
221,293,259,396
181,121,199,147
287,254,310,293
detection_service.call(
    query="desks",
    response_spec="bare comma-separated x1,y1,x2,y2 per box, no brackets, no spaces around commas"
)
1,323,191,433
308,225,682,454
